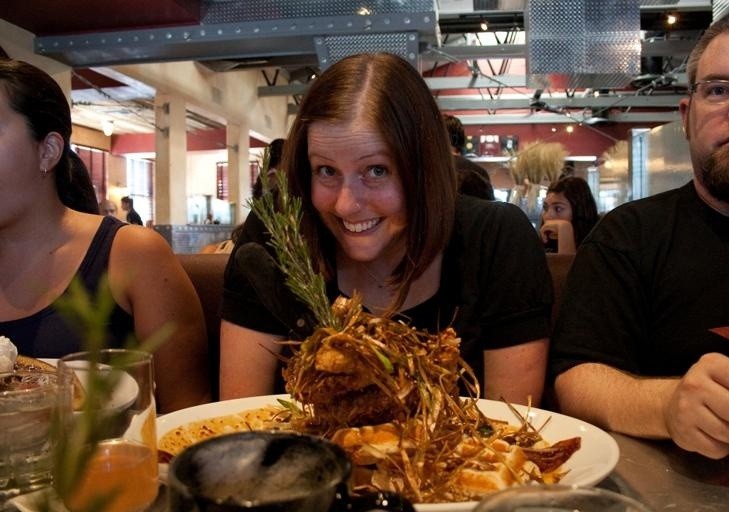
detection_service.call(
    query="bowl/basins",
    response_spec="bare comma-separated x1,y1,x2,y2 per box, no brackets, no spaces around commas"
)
471,484,651,512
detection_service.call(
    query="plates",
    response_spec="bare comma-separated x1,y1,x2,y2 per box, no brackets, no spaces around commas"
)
2,487,67,512
145,388,621,511
21,358,139,418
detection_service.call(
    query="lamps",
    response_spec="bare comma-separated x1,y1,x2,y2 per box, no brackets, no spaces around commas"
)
101,115,116,137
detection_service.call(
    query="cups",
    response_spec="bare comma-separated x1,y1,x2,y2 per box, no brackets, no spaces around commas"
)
0,369,72,495
165,431,416,512
51,349,159,512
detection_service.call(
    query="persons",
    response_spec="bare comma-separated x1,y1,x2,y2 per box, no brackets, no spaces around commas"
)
98,199,117,217
120,196,143,225
0,58,210,415
219,51,552,410
550,16,727,461
253,114,597,259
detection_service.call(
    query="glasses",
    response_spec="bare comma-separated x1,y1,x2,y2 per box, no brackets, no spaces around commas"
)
689,79,728,105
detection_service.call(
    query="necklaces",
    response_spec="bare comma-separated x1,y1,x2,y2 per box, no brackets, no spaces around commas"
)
357,262,421,289
